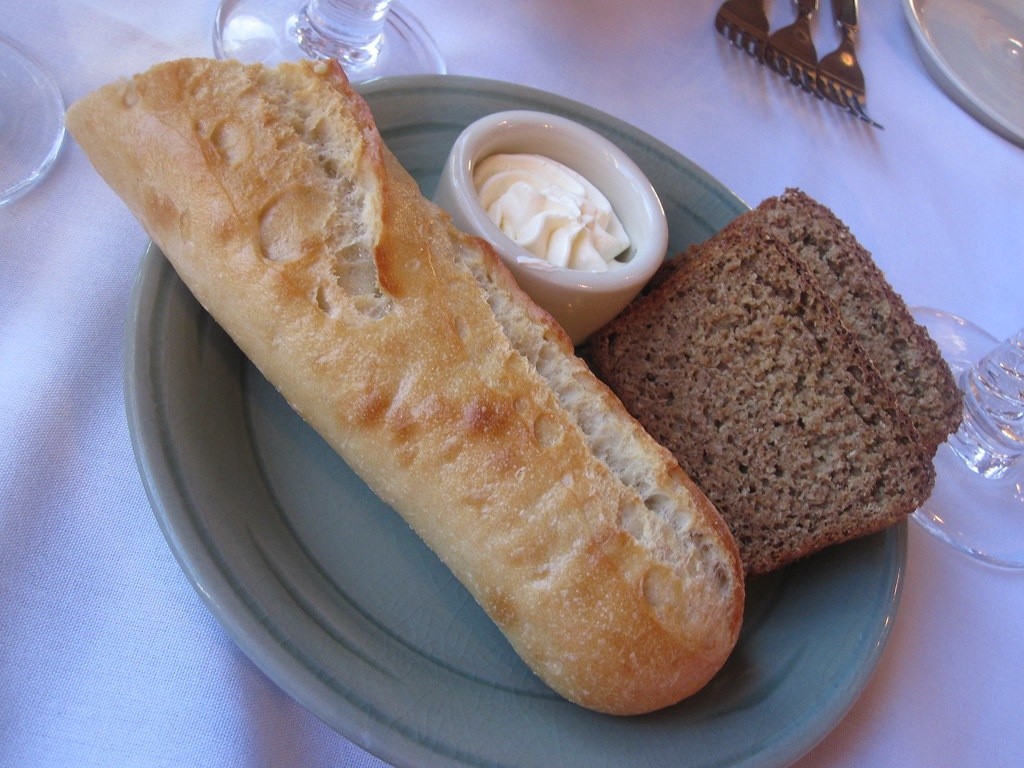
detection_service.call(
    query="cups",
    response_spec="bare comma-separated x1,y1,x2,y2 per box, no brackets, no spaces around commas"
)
429,109,669,346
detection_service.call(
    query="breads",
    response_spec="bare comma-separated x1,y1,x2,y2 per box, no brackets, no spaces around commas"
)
582,188,963,577
61,54,746,713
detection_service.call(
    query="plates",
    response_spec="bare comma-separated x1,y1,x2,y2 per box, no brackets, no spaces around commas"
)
903,2,1023,148
130,74,907,768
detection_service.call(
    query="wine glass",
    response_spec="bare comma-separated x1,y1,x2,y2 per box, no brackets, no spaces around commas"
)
2,46,64,207
908,304,1021,574
217,0,445,81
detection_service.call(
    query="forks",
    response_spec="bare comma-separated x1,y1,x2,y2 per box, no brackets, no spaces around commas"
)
769,2,826,100
716,1,770,66
817,0,884,129
